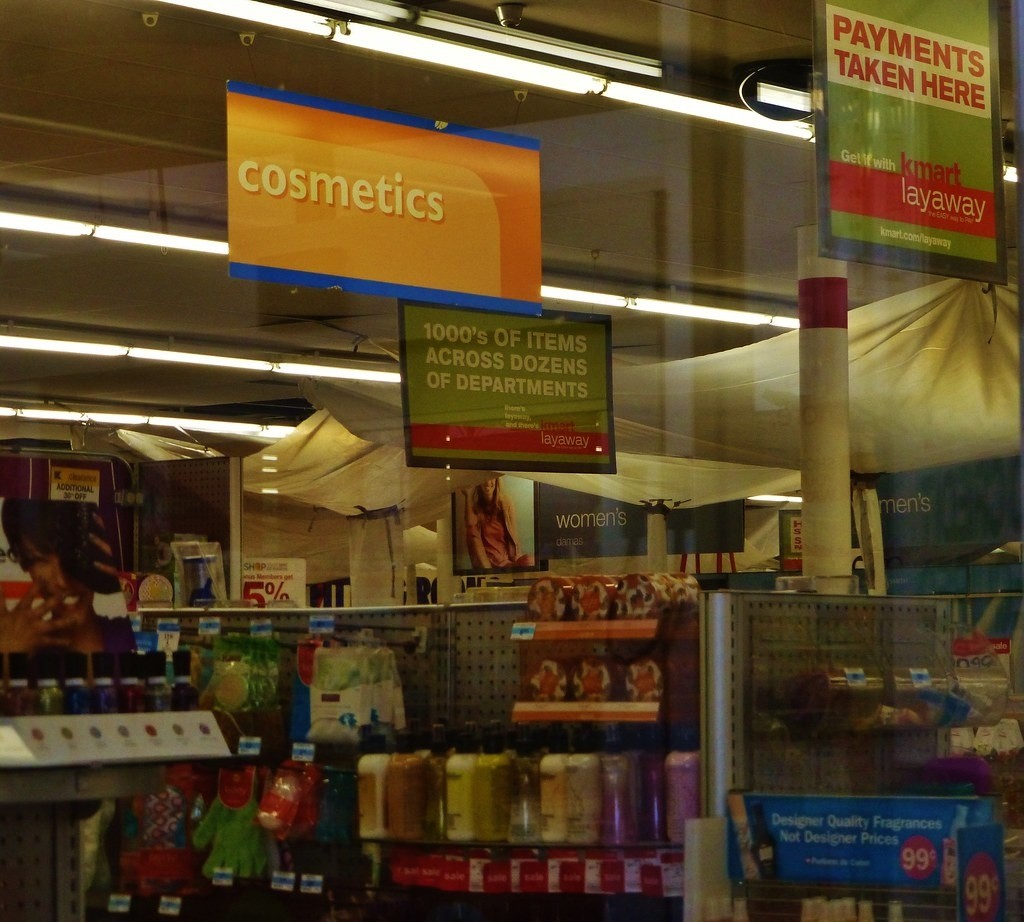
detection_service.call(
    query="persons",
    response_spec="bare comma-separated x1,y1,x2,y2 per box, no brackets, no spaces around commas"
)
1,499,137,653
461,471,531,569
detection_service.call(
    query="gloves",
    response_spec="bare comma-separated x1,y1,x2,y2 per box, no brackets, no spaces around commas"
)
194,767,270,881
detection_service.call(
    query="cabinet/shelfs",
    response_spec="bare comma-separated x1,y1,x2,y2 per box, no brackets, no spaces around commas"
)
0,447,1024,922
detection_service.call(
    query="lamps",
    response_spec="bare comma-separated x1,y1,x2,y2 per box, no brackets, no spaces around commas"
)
147,0,819,145
0,193,801,439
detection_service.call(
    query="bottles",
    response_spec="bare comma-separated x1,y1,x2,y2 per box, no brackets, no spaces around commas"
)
941,804,969,893
353,719,700,849
749,802,780,880
